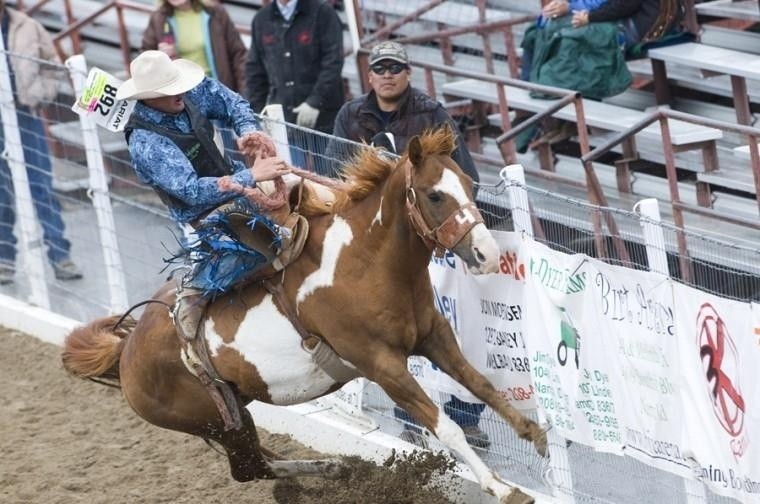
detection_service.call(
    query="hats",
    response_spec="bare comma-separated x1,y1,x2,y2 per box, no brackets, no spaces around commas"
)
367,38,410,67
115,48,206,103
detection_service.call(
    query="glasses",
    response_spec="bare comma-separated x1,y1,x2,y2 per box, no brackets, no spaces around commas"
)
370,61,405,74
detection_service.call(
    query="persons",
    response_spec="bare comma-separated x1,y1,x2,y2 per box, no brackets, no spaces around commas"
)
0,0,82,284
245,0,344,176
511,0,627,127
326,41,479,201
116,50,293,341
531,0,704,151
141,0,249,161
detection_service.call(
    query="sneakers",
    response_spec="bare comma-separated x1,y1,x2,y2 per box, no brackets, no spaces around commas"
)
53,258,82,280
1,263,15,282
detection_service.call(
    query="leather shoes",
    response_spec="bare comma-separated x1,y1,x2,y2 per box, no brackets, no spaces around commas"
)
397,426,430,445
462,424,490,447
172,271,212,342
547,121,591,145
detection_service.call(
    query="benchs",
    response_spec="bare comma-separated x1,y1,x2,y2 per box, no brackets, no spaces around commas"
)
0,0,760,227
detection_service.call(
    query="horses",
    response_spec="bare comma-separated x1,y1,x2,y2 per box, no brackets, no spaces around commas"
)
60,121,571,504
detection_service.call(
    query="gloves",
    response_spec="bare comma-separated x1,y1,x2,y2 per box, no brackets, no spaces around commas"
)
292,103,319,129
